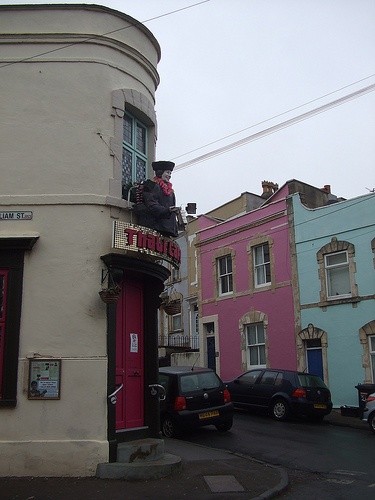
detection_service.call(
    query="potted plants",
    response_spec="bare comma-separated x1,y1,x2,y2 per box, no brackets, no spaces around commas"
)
262,180,278,194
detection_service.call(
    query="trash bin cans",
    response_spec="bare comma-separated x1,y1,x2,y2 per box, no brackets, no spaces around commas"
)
355,383,375,420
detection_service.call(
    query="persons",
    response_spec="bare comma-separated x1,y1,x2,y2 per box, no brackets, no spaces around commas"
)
135,159,179,238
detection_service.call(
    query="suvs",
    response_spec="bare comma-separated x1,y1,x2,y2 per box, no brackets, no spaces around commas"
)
160,366,236,438
223,368,333,423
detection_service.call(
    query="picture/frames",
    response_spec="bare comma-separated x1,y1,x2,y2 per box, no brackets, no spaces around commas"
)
27,358,61,400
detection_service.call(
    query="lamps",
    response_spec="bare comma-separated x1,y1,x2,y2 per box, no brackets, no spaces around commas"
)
185,203,197,214
